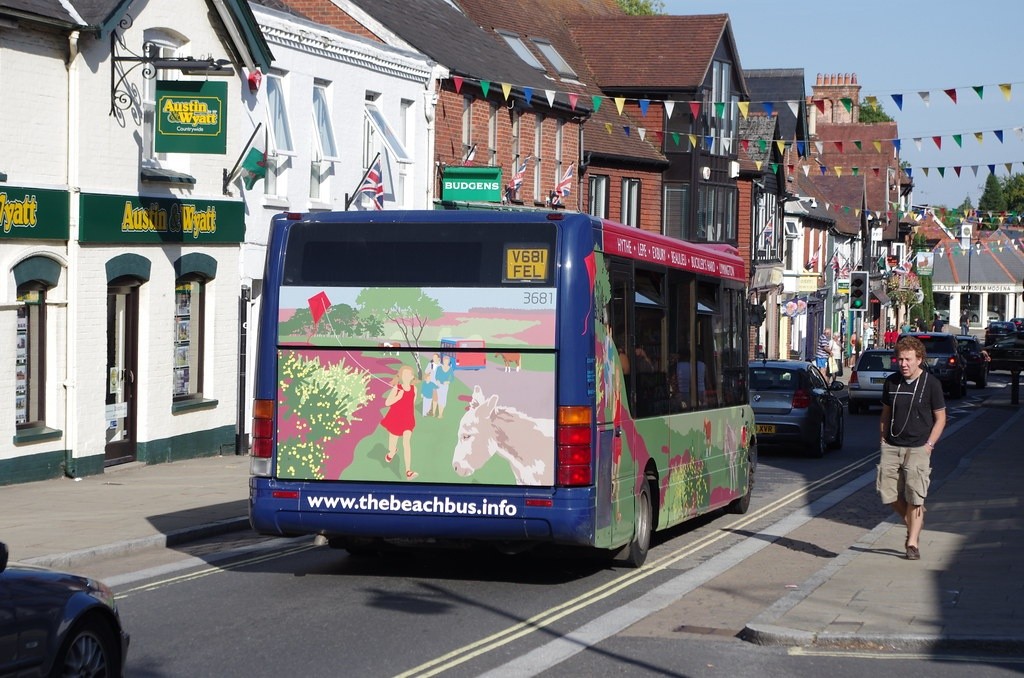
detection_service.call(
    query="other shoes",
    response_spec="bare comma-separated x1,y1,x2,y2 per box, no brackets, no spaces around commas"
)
906,545,920,561
904,532,919,549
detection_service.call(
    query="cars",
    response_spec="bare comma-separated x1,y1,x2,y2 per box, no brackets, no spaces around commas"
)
954,334,992,387
984,321,1018,347
932,301,1001,323
847,347,932,414
1009,318,1024,340
0,541,131,678
746,358,845,458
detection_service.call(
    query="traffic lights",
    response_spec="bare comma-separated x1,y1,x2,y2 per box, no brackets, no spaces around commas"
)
849,271,869,311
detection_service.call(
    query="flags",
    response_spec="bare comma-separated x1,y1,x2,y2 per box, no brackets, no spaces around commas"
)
762,214,774,245
238,124,267,191
504,154,532,203
545,163,573,207
808,247,913,282
463,144,477,167
354,154,383,211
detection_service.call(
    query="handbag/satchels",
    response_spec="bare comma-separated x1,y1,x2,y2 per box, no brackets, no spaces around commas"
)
829,355,839,374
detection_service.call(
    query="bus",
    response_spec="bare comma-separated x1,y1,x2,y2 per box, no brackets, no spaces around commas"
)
249,209,767,569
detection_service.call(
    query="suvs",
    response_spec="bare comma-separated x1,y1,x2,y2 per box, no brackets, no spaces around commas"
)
897,331,970,398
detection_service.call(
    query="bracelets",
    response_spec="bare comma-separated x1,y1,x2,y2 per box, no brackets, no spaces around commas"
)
926,439,936,449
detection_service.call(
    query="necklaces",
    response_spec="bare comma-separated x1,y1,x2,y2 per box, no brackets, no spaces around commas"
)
891,372,920,436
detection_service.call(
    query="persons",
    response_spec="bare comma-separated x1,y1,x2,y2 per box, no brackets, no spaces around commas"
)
876,336,947,561
816,313,945,384
960,311,969,335
